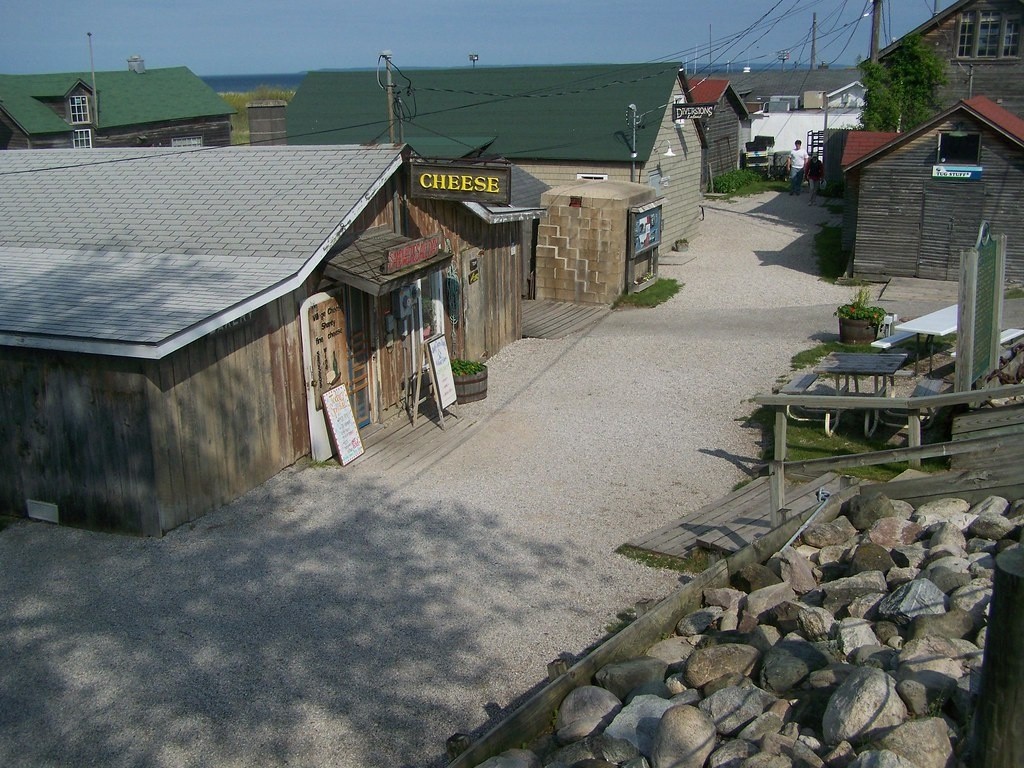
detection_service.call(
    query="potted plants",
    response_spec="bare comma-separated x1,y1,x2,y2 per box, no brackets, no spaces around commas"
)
675,237,689,252
833,277,887,346
634,272,656,293
450,357,488,404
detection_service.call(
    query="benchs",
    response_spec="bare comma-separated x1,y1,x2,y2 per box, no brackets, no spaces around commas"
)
882,379,944,431
952,328,1024,382
781,374,838,424
872,331,918,354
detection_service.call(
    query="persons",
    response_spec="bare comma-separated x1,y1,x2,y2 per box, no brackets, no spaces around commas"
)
787,139,809,196
804,155,825,207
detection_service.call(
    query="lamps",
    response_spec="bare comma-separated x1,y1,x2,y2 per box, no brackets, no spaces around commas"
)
949,122,968,136
653,139,676,157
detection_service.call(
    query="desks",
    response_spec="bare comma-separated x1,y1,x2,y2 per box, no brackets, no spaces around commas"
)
893,304,958,378
813,352,908,437
